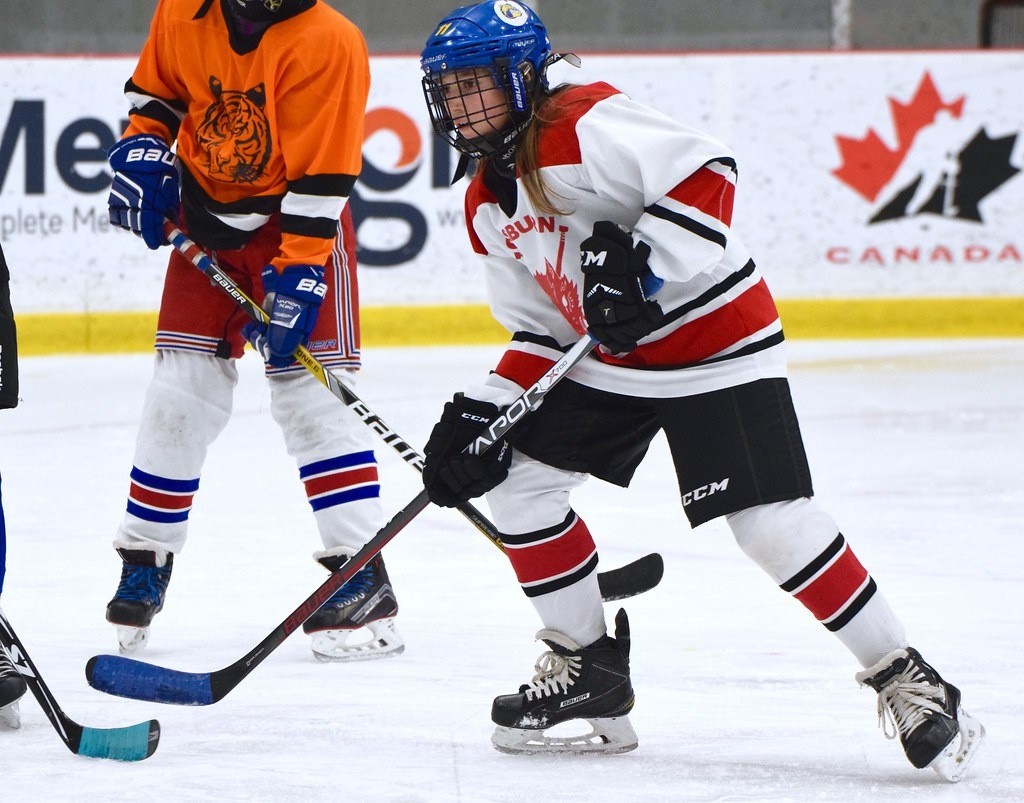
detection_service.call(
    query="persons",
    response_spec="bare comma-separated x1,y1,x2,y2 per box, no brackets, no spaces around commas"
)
0,246,30,729
106,0,401,662
422,0,985,784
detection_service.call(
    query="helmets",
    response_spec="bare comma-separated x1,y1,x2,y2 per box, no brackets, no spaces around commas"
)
421,0,551,159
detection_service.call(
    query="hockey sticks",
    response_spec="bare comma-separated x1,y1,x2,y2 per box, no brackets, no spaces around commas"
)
162,219,667,604
82,274,663,710
0,610,162,761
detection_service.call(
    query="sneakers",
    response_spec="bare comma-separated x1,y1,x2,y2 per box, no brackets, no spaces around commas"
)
105,540,174,655
0,639,26,728
491,607,639,754
854,646,985,783
305,545,405,660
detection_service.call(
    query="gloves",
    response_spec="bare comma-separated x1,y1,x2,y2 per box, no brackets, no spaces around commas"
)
105,134,182,250
422,391,515,507
579,221,663,355
240,264,329,368
